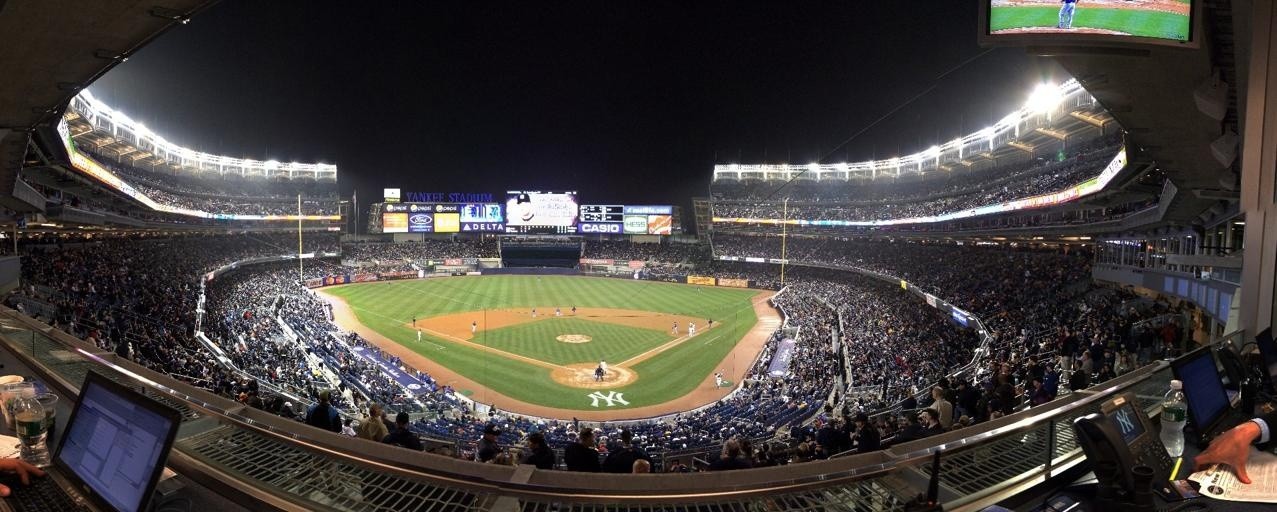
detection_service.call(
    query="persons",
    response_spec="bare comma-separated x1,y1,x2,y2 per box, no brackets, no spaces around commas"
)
1191,410,1271,485
1053,2,1080,28
2,460,42,499
2,134,1196,474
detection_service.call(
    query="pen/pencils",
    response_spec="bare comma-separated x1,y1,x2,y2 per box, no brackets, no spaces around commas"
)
1206,463,1221,476
1168,457,1183,481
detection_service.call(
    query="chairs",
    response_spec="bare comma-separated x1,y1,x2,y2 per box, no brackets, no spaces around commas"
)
231,323,975,512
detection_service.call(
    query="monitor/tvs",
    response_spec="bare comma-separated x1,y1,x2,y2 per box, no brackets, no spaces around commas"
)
977,0,1203,55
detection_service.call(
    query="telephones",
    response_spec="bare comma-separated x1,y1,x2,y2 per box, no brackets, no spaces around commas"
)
1073,391,1176,492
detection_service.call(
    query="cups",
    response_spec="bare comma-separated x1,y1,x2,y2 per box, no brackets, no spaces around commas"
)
38,390,61,437
0,374,26,384
1130,464,1154,507
0,380,34,429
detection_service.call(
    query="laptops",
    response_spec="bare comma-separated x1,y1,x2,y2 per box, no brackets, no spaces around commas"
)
1254,326,1277,366
0,368,182,512
1171,345,1262,449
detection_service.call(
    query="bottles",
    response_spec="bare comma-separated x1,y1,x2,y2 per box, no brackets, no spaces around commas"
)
14,389,51,466
1240,378,1256,414
1159,378,1186,458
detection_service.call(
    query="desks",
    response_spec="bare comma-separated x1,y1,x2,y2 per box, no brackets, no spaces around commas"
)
1,366,226,512
1035,399,1277,511
